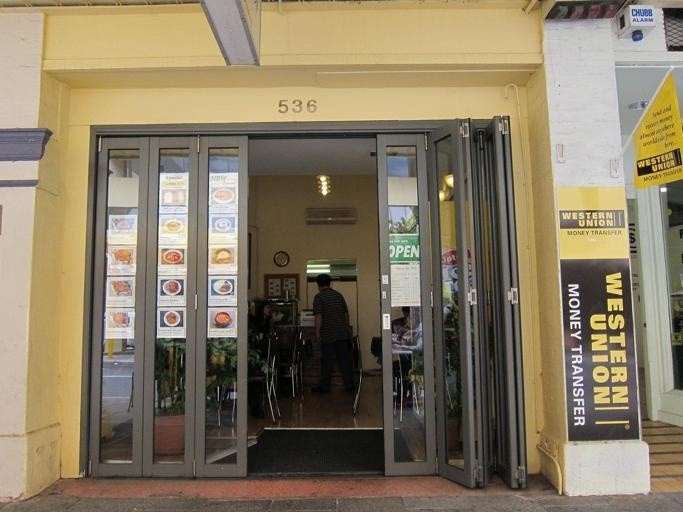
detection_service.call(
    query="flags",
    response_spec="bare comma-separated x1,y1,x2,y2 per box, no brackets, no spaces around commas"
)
632,75,683,189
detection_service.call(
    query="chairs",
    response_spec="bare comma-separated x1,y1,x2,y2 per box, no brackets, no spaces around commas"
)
353,335,402,416
213,328,306,428
445,333,456,415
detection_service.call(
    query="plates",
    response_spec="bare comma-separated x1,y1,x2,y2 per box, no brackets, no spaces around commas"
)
213,280,232,295
163,250,182,264
215,218,231,233
214,248,233,264
162,280,180,296
164,219,182,233
163,311,180,326
213,188,234,204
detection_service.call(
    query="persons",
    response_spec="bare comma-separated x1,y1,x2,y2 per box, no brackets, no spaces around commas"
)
313,273,358,392
254,303,272,331
392,306,419,334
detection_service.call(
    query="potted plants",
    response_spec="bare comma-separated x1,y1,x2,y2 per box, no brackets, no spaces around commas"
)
155,338,185,456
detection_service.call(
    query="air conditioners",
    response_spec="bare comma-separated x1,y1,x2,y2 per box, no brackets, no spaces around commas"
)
305,207,357,225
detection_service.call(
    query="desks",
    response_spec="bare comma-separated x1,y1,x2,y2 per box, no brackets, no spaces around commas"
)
392,350,424,416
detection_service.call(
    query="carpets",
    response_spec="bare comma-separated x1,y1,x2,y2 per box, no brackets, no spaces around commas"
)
214,429,414,470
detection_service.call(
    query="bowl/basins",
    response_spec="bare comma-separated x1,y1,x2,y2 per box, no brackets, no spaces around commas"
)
214,312,231,327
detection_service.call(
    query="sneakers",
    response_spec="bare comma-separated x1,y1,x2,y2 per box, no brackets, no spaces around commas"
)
311,383,360,394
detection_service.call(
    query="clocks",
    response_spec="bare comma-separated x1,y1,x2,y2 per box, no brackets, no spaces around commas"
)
273,251,290,266
